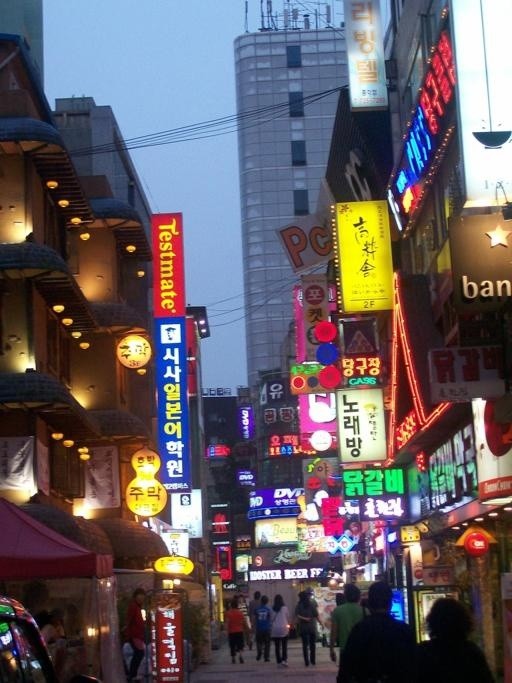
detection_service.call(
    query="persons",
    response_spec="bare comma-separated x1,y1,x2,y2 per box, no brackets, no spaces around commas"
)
293,590,325,665
250,595,273,662
412,595,499,683
270,594,290,667
334,591,348,608
246,590,262,651
333,581,419,681
120,586,147,682
302,586,318,616
329,583,365,671
221,599,246,663
39,602,83,648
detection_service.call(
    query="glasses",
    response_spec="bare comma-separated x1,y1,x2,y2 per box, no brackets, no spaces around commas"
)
46,180,147,462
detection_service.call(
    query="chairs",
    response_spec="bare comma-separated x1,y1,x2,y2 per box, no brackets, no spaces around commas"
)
232,658,244,664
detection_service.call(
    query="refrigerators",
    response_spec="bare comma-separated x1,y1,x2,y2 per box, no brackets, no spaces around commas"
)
278,660,288,666
